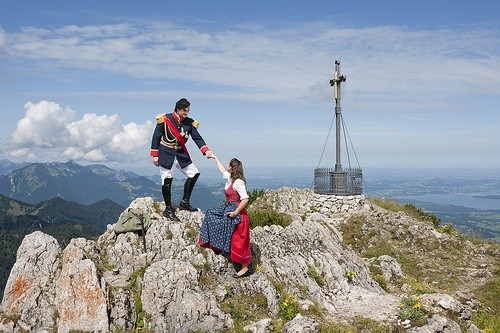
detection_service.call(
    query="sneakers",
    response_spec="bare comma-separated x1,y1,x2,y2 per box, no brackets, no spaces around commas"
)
177,201,198,212
163,208,180,221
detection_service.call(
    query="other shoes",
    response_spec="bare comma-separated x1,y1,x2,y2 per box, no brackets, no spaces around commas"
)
233,269,249,278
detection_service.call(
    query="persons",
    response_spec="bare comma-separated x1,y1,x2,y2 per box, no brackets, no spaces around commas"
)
197,151,252,278
151,99,214,222
